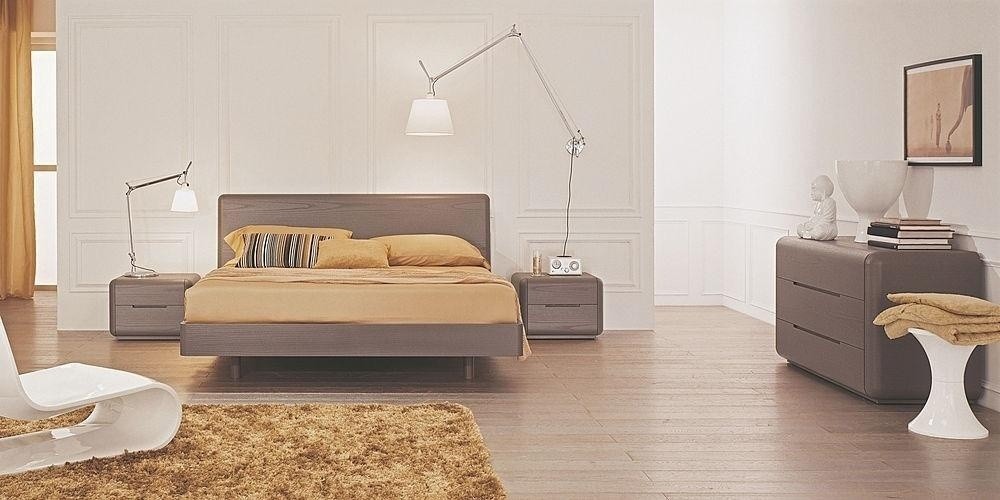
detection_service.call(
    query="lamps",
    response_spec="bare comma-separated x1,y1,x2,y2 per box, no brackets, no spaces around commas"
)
405,23,586,276
125,161,200,279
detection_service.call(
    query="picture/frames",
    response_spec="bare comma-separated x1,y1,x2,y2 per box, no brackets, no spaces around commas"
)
903,53,982,167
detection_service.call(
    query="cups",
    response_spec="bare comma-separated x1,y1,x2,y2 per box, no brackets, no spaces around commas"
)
532,245,542,276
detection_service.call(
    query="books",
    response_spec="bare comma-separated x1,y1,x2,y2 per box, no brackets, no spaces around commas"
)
866,217,955,251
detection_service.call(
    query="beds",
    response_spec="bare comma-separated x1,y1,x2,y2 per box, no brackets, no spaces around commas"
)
179,194,532,380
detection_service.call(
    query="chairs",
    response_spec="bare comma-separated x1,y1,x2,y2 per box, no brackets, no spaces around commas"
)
0,316,182,476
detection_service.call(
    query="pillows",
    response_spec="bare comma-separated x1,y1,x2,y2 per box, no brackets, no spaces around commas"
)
312,239,391,270
235,233,334,268
368,234,490,270
224,224,354,267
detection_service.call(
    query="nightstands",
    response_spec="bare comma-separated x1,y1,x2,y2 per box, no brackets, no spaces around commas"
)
109,273,201,341
511,272,604,341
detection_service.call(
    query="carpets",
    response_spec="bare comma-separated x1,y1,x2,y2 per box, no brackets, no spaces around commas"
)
0,400,509,500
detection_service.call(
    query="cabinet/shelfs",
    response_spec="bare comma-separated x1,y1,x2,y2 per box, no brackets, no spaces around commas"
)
775,235,986,406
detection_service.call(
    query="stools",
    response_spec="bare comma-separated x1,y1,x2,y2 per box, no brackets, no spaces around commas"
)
906,328,990,440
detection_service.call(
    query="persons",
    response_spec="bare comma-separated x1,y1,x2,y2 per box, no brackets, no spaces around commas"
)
796,175,838,239
